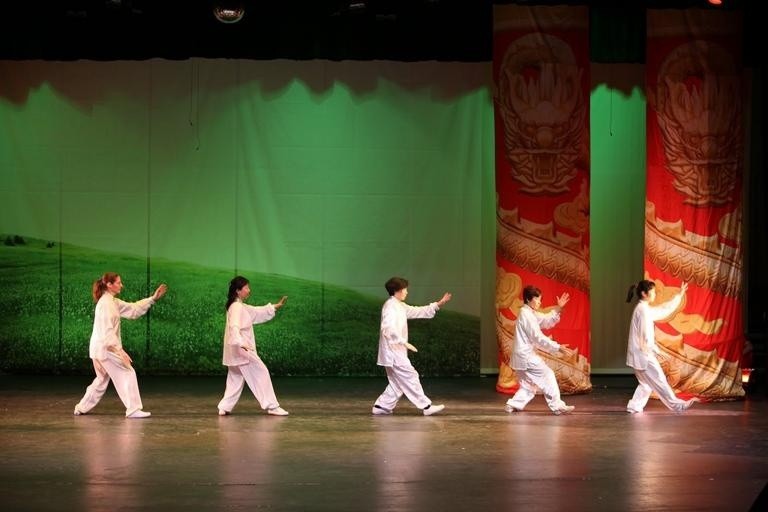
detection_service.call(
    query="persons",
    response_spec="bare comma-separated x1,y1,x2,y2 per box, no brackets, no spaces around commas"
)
71,270,170,418
215,275,293,417
624,278,697,413
503,284,575,415
367,277,453,416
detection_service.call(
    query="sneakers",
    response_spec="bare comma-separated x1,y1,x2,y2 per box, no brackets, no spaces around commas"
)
73,408,82,416
371,405,393,415
553,404,576,415
422,404,445,416
127,410,152,418
267,406,288,416
503,405,518,413
682,399,695,410
219,409,226,416
626,408,636,413
